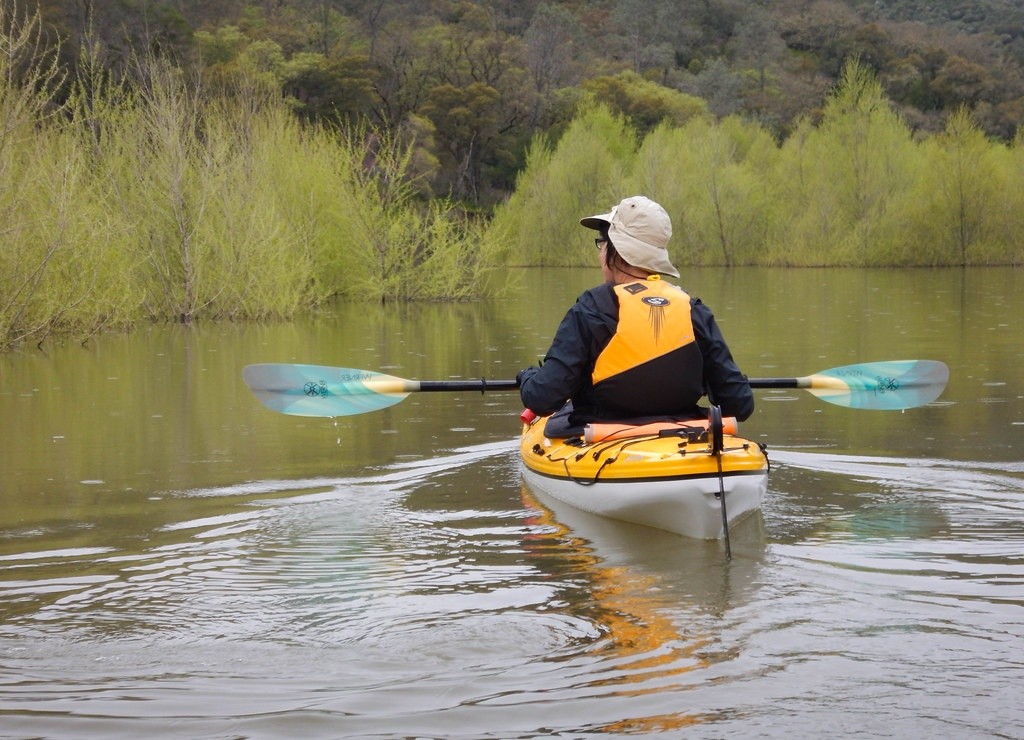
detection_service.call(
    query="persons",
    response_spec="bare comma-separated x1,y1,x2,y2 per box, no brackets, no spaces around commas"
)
513,196,755,428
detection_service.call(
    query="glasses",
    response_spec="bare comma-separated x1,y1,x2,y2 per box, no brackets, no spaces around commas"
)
595,237,609,249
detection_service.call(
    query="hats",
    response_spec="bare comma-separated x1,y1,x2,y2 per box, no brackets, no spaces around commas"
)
579,196,681,278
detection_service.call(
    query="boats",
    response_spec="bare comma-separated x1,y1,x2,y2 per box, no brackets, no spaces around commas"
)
516,397,770,541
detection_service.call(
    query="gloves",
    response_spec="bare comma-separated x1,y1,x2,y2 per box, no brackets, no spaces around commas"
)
516,365,539,385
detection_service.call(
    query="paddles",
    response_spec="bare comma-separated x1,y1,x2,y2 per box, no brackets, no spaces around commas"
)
242,357,952,418
